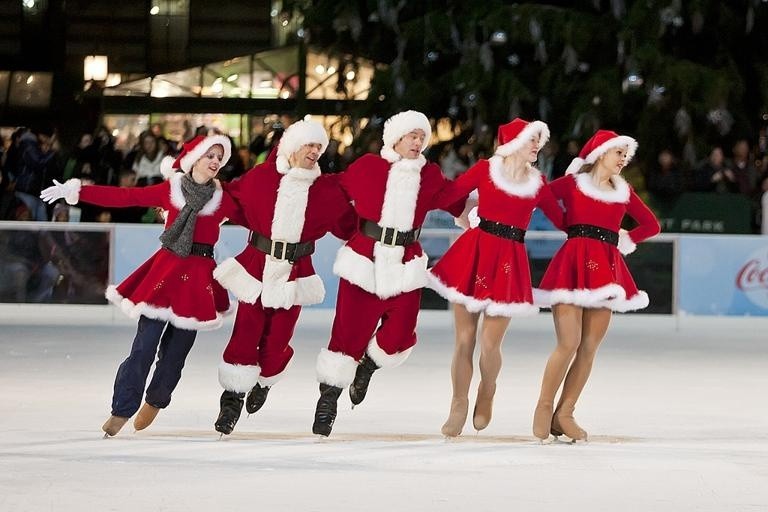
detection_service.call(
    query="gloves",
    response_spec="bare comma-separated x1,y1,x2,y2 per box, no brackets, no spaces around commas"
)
40,179,65,205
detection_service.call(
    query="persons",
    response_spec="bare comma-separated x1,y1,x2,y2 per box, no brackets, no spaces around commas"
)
533,130,660,441
428,118,566,436
214,120,356,436
302,3,768,233
38,134,249,437
309,110,478,435
2,128,285,303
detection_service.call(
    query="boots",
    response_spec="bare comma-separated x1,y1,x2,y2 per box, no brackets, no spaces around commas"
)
215,390,245,435
313,383,343,436
349,352,380,405
246,383,270,413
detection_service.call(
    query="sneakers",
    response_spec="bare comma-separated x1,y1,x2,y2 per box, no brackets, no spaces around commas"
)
103,415,129,436
550,411,587,440
442,396,468,436
533,404,553,439
473,382,496,430
134,403,159,430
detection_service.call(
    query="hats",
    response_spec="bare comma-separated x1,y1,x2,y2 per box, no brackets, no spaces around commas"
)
383,110,432,153
579,130,639,173
495,118,551,157
160,135,231,178
266,120,330,160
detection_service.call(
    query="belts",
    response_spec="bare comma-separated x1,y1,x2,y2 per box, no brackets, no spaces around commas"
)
360,219,421,248
251,232,314,262
